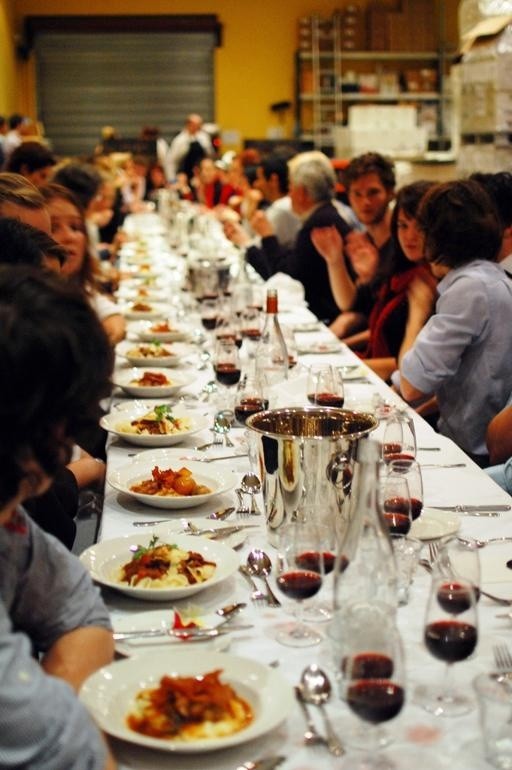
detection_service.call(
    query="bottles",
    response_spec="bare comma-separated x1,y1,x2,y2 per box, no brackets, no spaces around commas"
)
332,441,399,665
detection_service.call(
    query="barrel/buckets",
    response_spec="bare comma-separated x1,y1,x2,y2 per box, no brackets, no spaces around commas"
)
244,404,380,555
189,256,231,299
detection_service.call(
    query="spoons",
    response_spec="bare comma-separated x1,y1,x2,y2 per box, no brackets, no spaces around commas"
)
114,604,245,638
247,549,282,608
212,412,235,447
130,506,235,526
301,664,345,759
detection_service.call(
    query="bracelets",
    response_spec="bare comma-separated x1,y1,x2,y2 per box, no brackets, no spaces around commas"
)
96,458,104,464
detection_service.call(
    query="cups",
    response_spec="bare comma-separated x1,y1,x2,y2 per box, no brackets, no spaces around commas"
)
390,531,425,607
256,287,291,398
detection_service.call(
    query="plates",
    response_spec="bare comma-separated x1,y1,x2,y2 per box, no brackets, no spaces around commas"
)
106,366,186,397
77,647,294,753
104,459,233,510
110,223,191,367
74,532,240,600
97,409,205,449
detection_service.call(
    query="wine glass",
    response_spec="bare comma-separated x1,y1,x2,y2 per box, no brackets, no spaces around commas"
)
232,372,269,487
338,600,410,769
384,474,412,541
411,577,479,719
385,416,419,475
213,346,242,410
394,455,425,526
313,369,345,408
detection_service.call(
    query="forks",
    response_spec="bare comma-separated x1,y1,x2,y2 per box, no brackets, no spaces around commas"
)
293,689,329,760
237,565,266,609
196,439,223,450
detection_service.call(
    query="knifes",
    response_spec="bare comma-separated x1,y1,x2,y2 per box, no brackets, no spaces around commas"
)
429,504,511,515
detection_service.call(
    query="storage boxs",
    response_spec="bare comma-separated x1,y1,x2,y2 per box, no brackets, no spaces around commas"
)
365,2,438,51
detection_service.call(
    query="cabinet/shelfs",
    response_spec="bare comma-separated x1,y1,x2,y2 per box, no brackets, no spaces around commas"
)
284,50,450,152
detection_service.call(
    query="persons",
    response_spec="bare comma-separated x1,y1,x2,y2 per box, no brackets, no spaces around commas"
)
1,276,116,769
397,179,510,469
0,112,164,551
164,112,442,430
478,404,511,487
